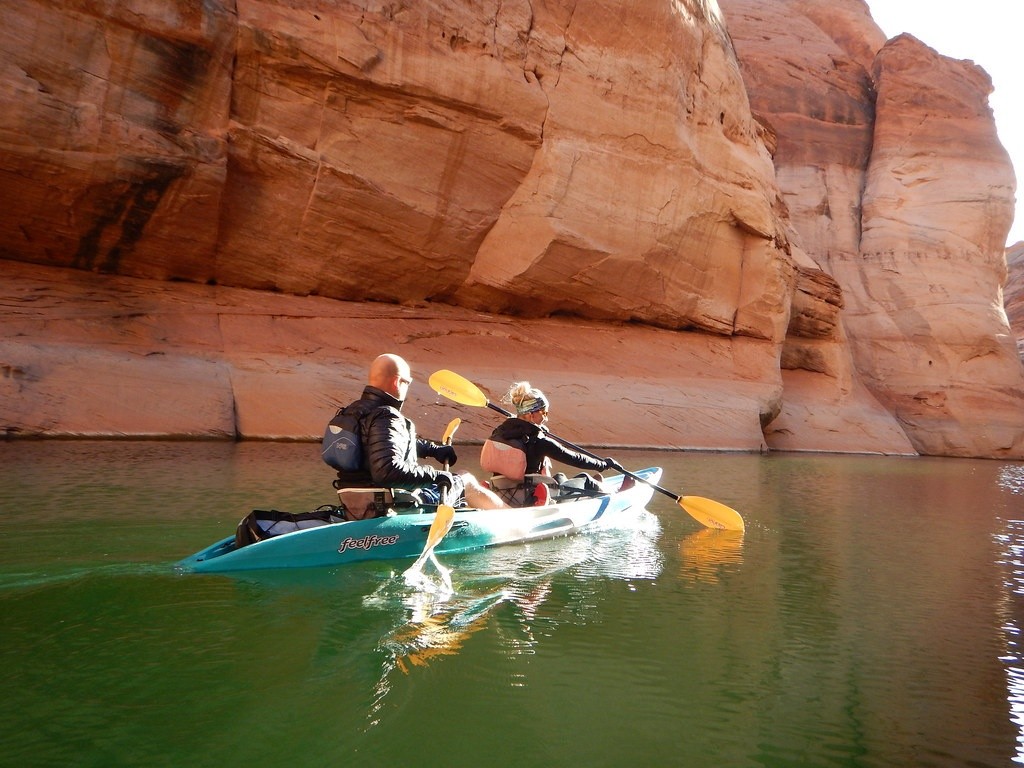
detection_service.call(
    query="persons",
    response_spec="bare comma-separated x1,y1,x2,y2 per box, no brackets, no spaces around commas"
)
322,354,512,516
481,381,635,505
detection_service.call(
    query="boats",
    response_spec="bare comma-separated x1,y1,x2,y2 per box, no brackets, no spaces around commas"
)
171,464,665,576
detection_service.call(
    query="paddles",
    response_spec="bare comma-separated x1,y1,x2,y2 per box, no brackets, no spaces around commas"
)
427,370,746,530
418,419,464,559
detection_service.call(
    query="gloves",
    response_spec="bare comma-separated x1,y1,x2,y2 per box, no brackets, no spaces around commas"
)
434,470,453,492
434,445,458,467
604,457,623,470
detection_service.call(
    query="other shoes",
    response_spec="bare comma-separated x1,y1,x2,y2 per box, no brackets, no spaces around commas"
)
595,474,605,483
620,475,635,492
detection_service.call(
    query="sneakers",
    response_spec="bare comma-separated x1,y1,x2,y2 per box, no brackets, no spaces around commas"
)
524,482,550,506
